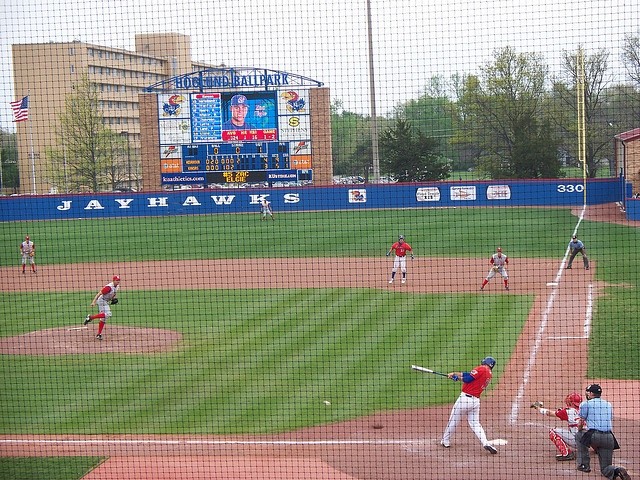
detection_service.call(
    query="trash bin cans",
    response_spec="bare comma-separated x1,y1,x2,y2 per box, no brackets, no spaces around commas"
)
625,197,640,221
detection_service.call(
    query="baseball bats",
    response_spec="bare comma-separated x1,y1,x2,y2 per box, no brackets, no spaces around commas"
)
411,365,458,382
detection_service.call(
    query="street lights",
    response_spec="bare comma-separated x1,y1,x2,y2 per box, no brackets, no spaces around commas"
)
121,132,130,193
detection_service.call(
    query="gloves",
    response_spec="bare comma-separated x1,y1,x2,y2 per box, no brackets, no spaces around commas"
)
411,254,414,259
452,375,459,382
386,251,390,256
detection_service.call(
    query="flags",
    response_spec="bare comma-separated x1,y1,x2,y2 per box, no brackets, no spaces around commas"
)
9,96,28,123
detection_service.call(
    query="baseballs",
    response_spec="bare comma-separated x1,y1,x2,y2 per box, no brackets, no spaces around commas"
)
539,402,544,407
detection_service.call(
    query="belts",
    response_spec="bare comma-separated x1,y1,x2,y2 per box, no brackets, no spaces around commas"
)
466,394,479,398
396,254,406,257
590,429,610,434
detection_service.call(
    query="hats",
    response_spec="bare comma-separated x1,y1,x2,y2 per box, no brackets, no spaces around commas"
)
497,247,502,252
573,235,577,239
113,276,120,281
231,95,249,107
25,236,29,240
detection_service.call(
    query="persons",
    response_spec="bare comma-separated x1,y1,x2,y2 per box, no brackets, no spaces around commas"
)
566,236,589,269
20,236,37,274
574,384,632,480
83,275,120,340
440,356,497,454
386,235,413,284
480,247,510,291
261,199,275,221
222,95,256,130
534,393,597,461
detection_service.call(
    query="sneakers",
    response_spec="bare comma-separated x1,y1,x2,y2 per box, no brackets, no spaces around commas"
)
96,333,102,339
402,280,405,283
32,270,36,273
556,451,575,461
484,443,497,454
577,464,591,473
480,287,483,290
617,467,631,480
506,287,509,291
84,314,92,325
567,266,571,269
586,267,589,270
441,439,450,448
389,281,392,283
22,271,25,273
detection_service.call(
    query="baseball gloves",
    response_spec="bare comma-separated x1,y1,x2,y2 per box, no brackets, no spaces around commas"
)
30,252,35,257
111,299,117,305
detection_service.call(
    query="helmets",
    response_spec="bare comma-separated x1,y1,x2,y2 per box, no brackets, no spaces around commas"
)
399,235,404,240
585,384,602,400
481,357,496,369
564,393,583,406
